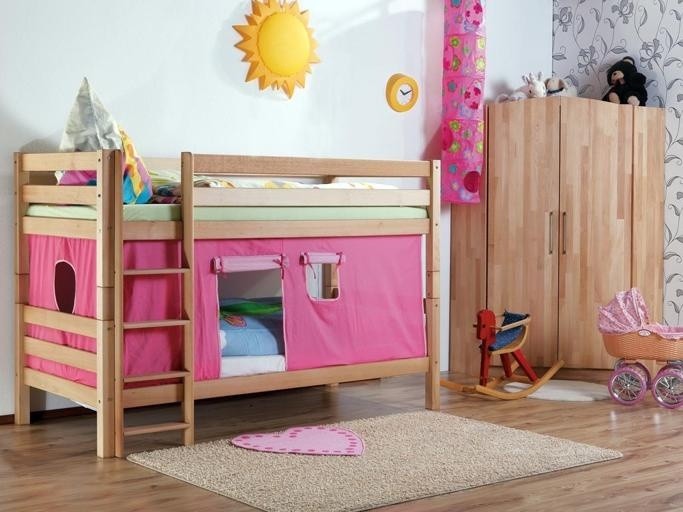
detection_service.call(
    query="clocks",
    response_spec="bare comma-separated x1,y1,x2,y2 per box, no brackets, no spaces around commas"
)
386,75,418,113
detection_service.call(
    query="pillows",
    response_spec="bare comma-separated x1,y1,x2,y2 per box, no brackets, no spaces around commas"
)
54,78,152,204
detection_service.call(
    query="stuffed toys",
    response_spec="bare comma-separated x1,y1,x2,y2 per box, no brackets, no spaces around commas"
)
493,72,568,103
601,56,648,107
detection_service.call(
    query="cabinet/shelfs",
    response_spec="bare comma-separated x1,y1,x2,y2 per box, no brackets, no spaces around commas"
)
447,98,666,380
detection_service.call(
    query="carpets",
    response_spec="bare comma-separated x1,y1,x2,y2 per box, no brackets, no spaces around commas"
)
121,408,623,510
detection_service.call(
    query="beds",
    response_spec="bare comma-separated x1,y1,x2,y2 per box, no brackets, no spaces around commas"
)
13,152,441,460
